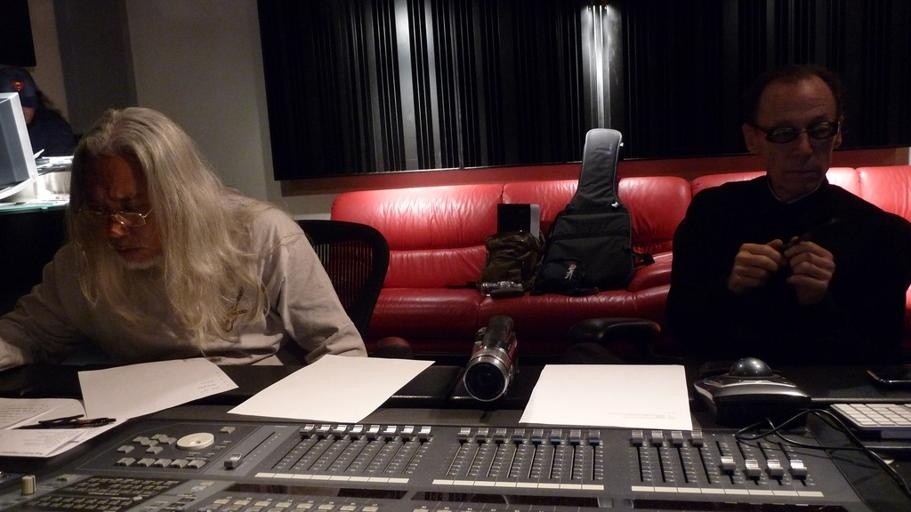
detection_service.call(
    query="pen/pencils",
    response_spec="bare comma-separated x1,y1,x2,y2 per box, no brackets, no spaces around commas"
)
15,414,117,429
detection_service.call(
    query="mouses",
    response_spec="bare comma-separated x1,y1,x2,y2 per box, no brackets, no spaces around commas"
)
727,356,774,378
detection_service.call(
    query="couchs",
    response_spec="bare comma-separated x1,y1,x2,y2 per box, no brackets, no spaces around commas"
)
331,147,911,355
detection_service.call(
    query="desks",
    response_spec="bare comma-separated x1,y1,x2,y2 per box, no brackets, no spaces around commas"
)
1,345,911,425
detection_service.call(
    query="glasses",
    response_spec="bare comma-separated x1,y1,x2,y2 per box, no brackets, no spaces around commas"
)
72,206,153,228
753,122,840,144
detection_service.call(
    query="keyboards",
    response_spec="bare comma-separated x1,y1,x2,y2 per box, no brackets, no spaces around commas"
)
829,403,911,439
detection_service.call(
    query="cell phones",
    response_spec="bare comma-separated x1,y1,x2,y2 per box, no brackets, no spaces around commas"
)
864,367,910,389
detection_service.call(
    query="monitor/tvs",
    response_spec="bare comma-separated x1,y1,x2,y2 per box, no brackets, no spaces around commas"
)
0,92,39,198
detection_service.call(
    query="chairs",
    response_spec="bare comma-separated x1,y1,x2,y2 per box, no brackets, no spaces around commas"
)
280,220,390,349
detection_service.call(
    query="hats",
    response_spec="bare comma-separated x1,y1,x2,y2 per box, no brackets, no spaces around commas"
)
1,67,39,109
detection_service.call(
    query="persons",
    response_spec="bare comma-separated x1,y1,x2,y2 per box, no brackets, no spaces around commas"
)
0,105,369,376
665,68,911,384
1,66,75,245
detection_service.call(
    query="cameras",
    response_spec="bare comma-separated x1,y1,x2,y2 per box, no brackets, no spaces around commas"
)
463,314,518,403
